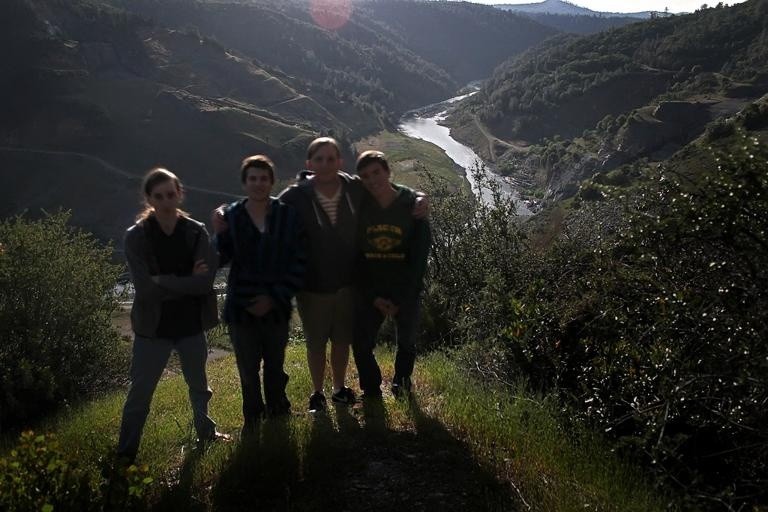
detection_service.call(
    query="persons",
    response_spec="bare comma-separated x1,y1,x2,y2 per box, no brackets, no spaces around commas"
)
352,150,432,401
209,138,430,412
112,167,232,463
216,155,293,444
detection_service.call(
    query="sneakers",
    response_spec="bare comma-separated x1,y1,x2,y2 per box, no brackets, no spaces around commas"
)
308,391,327,413
331,386,361,405
211,431,234,445
